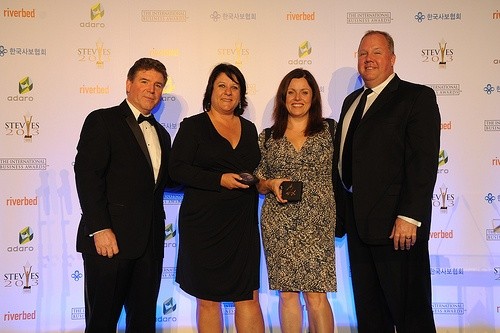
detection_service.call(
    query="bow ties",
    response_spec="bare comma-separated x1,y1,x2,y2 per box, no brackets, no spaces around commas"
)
138,113,154,126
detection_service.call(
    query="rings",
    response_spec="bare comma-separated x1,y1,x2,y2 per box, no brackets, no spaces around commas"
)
406,237,412,239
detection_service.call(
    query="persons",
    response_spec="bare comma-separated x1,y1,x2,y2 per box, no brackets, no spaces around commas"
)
253,67,340,333
330,29,441,333
168,62,265,333
73,56,171,333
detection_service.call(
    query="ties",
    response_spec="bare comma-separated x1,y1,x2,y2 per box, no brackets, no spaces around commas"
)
341,87,374,189
